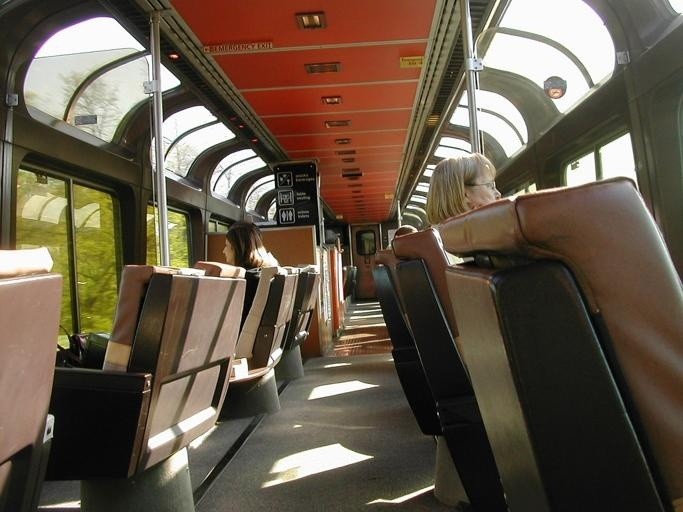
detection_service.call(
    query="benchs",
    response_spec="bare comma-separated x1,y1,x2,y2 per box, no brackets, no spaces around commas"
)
49,260,249,510
369,176,683,512
273,262,322,381
1,240,65,510
217,262,302,424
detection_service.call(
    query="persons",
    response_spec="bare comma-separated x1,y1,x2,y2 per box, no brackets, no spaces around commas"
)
424,150,503,227
221,219,279,350
